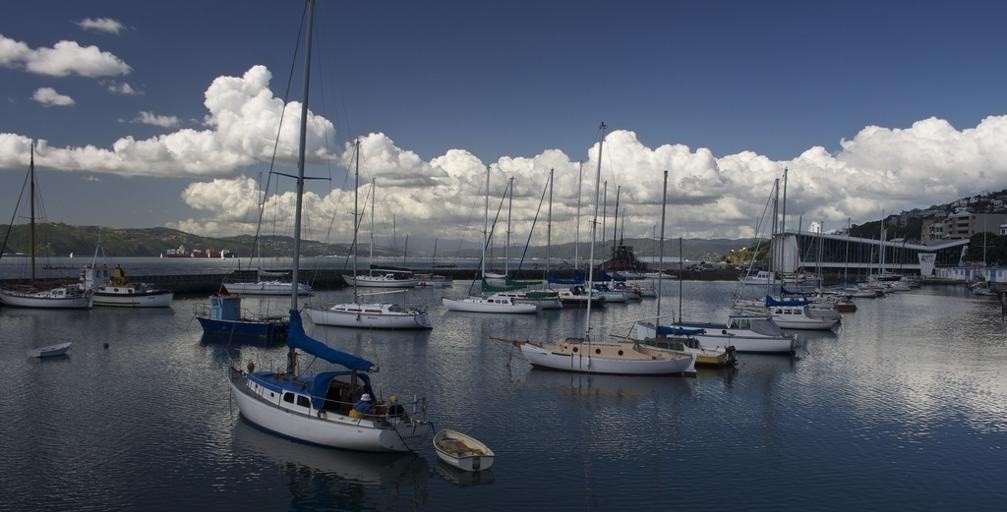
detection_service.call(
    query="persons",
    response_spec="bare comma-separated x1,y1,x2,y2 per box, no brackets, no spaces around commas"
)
352,393,404,421
113,264,127,285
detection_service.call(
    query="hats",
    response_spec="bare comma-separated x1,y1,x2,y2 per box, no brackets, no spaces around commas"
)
390,394,399,402
360,393,372,402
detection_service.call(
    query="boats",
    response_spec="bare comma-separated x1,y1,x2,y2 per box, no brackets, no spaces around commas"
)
668,169,912,354
433,424,494,471
0,142,175,308
26,342,72,358
